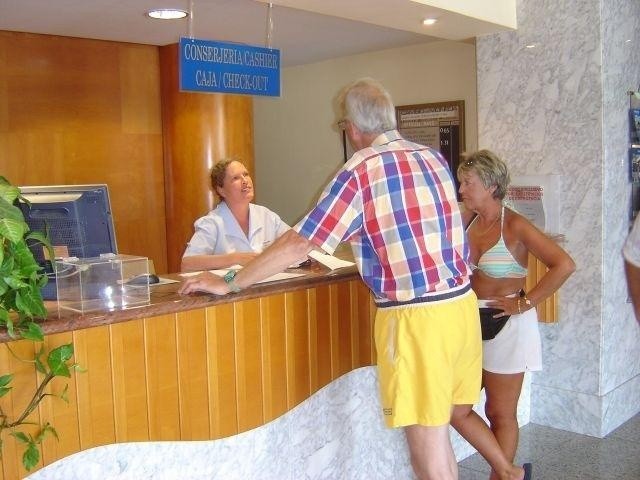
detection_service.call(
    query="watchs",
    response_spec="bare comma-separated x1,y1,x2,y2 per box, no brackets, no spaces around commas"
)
522,295,536,309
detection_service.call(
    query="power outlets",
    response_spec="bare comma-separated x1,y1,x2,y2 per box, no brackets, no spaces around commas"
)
397,103,467,201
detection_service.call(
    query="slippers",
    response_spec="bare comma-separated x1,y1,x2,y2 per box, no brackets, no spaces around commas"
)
522,462,533,480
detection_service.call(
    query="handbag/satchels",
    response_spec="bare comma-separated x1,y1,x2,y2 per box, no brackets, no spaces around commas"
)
479,307,511,340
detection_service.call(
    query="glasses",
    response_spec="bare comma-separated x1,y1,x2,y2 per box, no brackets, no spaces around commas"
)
462,158,485,176
336,119,347,130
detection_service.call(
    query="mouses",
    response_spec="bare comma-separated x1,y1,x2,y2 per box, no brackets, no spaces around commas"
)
125,273,160,285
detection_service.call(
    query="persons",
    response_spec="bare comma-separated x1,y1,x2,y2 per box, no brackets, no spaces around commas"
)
619,209,639,331
179,157,293,274
455,146,578,480
177,76,484,479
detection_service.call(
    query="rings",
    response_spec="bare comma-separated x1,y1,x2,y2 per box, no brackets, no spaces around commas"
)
501,311,505,317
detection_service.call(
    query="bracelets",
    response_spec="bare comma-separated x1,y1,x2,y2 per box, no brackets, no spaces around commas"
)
516,298,522,316
225,270,243,294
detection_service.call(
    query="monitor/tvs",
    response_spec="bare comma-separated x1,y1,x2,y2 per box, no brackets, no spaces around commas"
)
16,183,123,301
